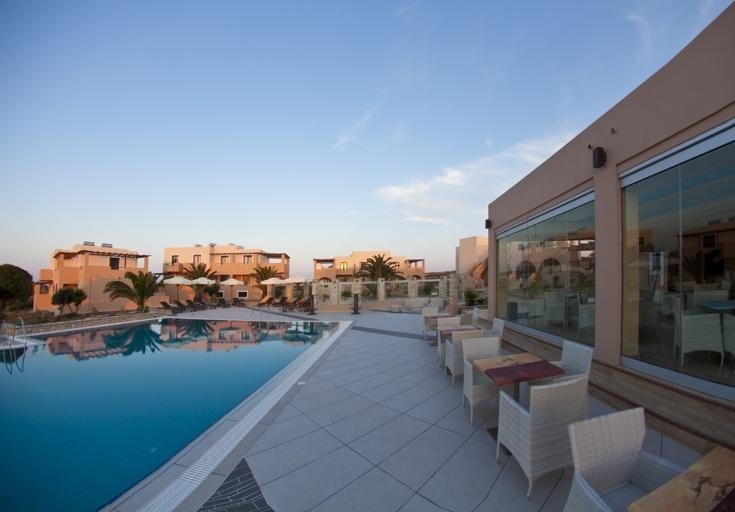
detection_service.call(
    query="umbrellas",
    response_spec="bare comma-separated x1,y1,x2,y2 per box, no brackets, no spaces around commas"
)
285,274,306,298
163,275,192,303
260,277,286,297
220,278,244,298
191,277,216,297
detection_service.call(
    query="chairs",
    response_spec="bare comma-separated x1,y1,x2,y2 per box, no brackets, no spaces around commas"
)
256,296,313,314
559,405,684,511
420,306,507,386
493,371,591,498
526,286,596,336
654,280,735,365
536,340,597,385
460,335,510,426
159,297,245,314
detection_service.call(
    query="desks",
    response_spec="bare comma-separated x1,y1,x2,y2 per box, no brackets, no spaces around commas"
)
471,352,564,456
627,444,735,511
